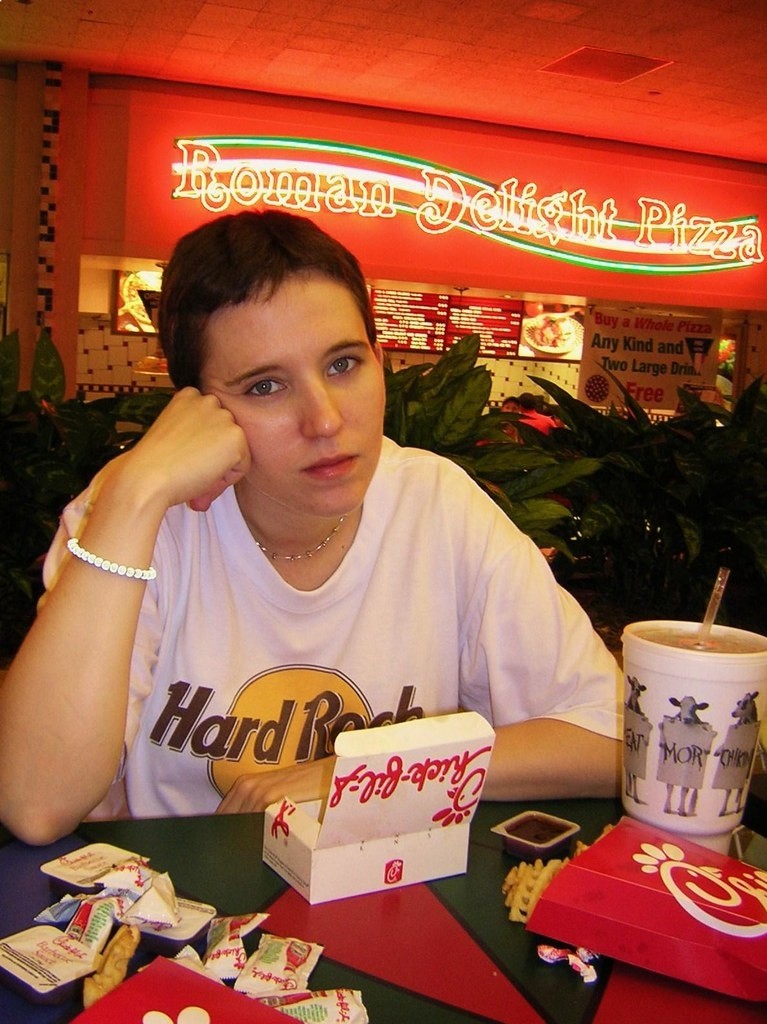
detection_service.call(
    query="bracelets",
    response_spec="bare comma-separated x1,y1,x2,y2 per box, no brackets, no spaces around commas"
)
68,538,156,579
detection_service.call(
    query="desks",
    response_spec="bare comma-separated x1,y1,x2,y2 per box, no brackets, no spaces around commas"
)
1,803,767,1024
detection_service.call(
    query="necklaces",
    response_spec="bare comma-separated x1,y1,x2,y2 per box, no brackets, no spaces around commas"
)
257,514,347,560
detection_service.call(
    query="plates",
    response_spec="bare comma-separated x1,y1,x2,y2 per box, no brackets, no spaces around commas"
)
524,314,586,356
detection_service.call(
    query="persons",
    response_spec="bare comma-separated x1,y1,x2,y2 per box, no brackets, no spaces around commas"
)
477,393,564,445
0,209,628,846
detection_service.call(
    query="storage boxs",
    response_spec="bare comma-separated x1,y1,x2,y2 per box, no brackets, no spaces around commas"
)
262,710,496,906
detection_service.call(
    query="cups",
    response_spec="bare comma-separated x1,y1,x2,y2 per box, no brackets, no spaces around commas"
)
622,620,767,858
